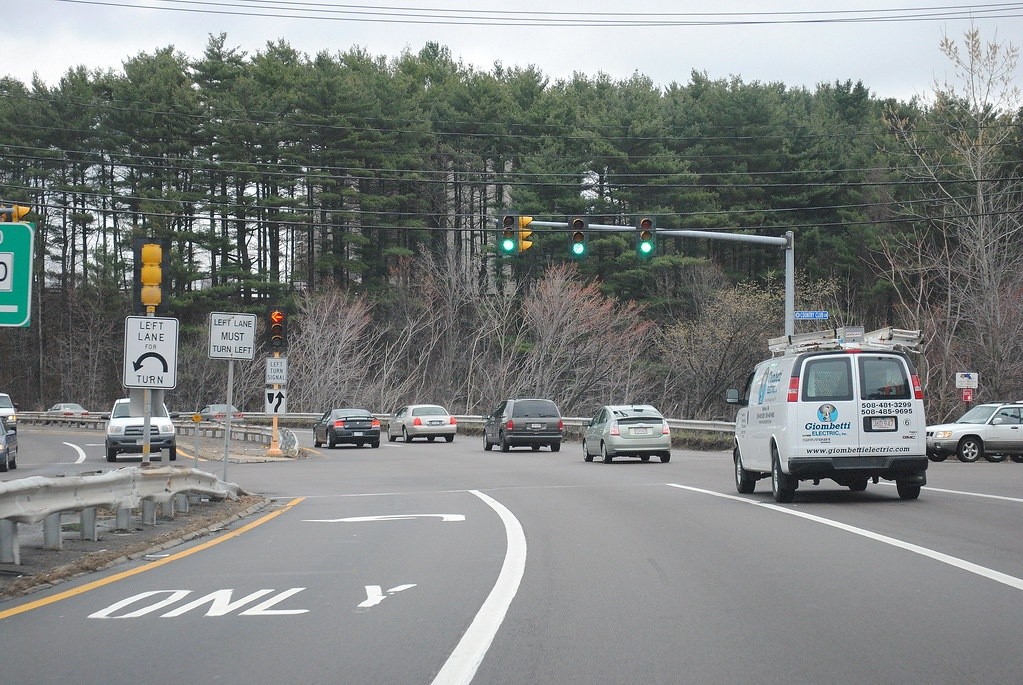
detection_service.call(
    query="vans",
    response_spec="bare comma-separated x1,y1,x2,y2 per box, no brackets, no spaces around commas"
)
0,393,19,436
725,326,929,505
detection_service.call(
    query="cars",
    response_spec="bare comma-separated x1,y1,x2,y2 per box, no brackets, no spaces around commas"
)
581,404,672,464
0,417,19,472
925,400,1023,463
197,403,244,427
313,408,380,449
483,398,564,453
387,404,457,443
43,402,90,425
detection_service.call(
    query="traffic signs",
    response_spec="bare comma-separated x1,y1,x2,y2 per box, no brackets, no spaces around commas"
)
0,220,36,328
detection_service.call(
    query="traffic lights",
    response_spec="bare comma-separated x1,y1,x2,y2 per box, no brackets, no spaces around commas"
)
11,204,31,224
567,216,589,261
518,216,533,253
496,209,519,254
635,215,658,258
265,306,288,352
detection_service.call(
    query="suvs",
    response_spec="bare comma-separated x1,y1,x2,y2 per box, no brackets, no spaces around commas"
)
101,398,180,462
